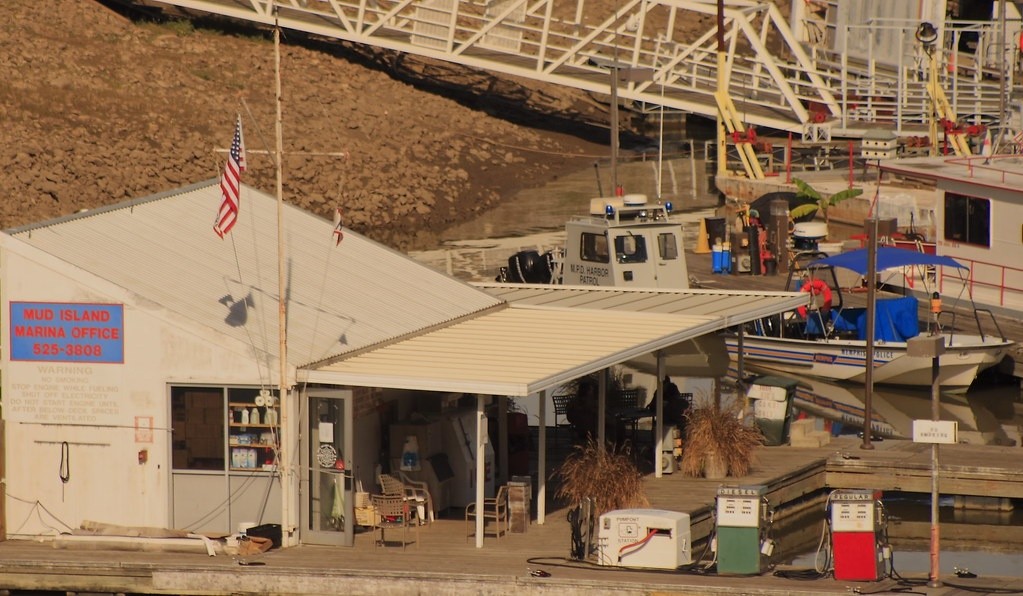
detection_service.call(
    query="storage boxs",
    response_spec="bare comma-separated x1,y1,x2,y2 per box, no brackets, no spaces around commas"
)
174,392,223,467
262,465,277,472
507,475,533,533
354,492,370,508
353,505,382,527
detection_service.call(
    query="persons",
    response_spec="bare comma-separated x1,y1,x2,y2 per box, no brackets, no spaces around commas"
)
646,375,686,457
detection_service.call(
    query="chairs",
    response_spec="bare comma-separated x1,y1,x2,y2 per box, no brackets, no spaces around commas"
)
552,394,579,446
616,387,641,444
398,471,436,526
377,473,431,530
368,492,420,552
464,485,511,543
680,392,693,411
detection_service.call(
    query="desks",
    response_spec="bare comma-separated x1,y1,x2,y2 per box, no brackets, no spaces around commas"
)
618,408,657,443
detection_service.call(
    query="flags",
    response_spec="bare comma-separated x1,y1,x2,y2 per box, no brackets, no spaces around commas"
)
212,115,247,242
332,175,343,246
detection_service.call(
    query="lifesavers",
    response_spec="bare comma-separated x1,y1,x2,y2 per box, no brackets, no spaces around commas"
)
797,279,833,321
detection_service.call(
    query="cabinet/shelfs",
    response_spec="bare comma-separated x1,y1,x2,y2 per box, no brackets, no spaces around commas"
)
227,391,281,471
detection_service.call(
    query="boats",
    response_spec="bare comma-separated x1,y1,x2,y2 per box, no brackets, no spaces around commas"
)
717,361,1023,446
496,11,1019,393
